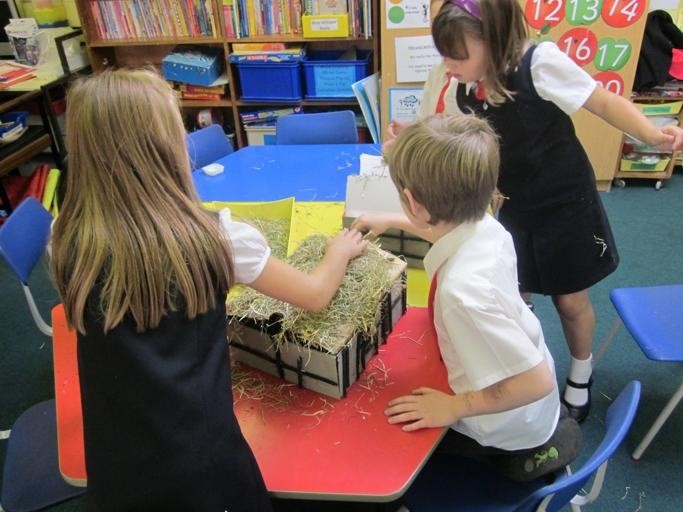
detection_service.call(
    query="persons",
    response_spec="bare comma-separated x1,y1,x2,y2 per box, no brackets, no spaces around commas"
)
347,108,587,511
45,65,368,511
384,0,683,427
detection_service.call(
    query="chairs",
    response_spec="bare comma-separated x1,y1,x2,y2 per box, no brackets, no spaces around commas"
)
0,399,89,512
397,380,642,512
0,197,55,338
593,284,683,461
185,124,235,172
275,109,358,144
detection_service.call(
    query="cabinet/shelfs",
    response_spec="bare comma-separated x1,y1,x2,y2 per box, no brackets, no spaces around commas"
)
0,0,379,224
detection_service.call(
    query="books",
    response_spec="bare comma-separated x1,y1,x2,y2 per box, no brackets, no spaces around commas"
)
217,1,379,38
230,43,305,61
86,2,218,39
237,107,311,133
348,70,382,148
146,46,232,103
0,59,39,93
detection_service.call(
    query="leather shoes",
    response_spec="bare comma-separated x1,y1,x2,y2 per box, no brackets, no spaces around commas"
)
562,376,594,424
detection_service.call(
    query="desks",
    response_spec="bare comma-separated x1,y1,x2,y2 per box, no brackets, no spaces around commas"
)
49,143,454,504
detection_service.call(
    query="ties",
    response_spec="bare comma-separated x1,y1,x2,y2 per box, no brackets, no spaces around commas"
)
427,274,448,375
474,79,485,100
